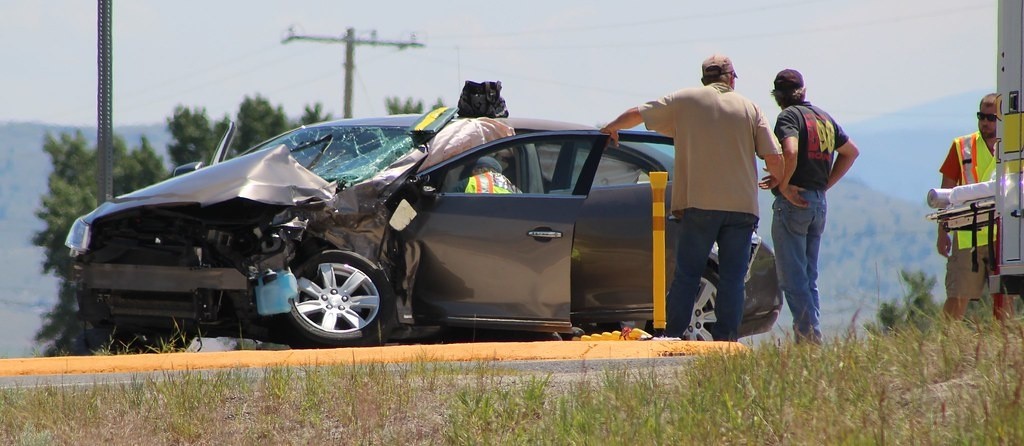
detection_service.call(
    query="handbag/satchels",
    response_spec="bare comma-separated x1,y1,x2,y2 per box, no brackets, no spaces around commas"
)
457,80,509,118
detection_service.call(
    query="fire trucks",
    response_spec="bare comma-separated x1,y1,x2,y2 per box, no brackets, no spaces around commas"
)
926,0,1024,303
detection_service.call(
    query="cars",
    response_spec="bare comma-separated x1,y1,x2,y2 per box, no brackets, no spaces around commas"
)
65,110,783,350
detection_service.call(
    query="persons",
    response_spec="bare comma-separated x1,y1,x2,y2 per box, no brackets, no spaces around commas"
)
602,55,785,344
937,93,998,323
771,69,860,343
449,156,521,194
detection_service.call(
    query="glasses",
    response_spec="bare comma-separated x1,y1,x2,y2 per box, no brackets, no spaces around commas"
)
977,112,996,122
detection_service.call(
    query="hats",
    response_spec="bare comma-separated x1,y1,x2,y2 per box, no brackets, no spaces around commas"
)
773,69,804,91
472,156,502,173
702,54,738,79
